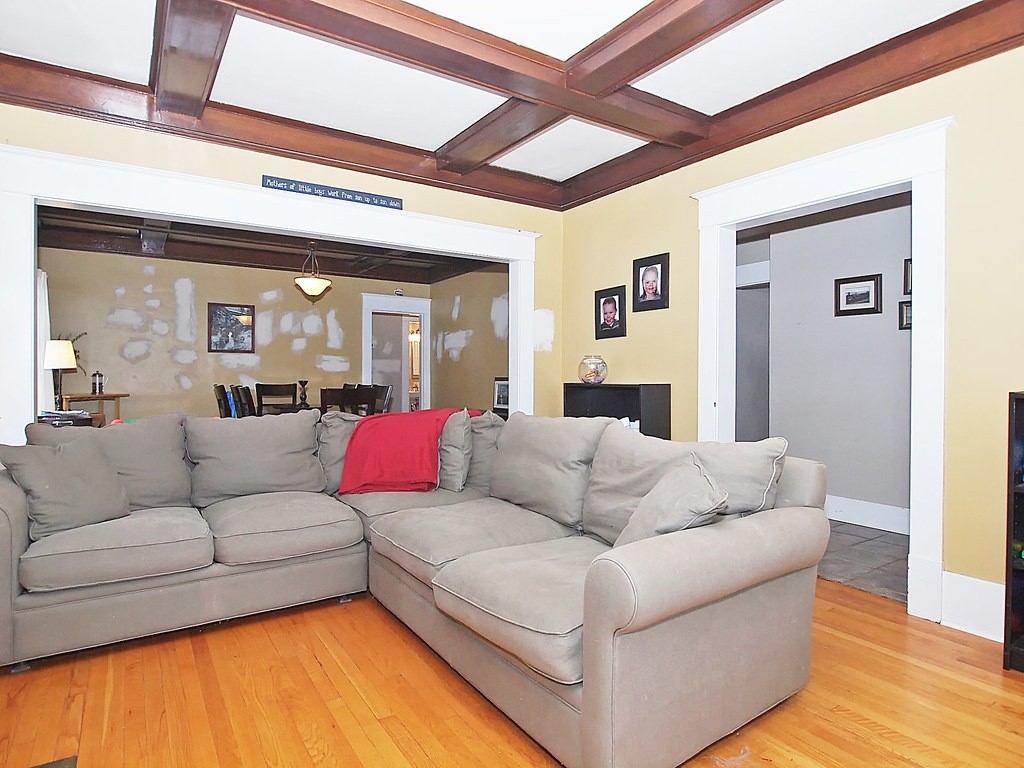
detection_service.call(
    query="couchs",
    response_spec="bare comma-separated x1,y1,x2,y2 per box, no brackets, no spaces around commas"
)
0,410,830,768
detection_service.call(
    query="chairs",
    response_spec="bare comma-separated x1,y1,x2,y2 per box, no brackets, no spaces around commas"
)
213,382,393,417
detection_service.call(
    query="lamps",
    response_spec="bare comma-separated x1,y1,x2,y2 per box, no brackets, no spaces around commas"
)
41,339,77,410
295,241,331,297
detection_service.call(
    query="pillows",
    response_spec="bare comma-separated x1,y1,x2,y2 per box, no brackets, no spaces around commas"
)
488,412,618,531
182,408,328,505
613,453,727,548
438,406,473,492
313,410,366,493
465,411,506,494
0,431,132,541
583,420,789,545
24,413,191,510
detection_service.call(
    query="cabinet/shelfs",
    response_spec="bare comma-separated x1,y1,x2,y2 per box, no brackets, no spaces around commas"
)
563,383,671,441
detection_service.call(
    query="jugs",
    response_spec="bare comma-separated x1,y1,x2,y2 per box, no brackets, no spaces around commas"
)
90,371,108,395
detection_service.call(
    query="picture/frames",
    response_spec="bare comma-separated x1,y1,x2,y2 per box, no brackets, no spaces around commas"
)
834,259,912,330
594,285,626,339
633,253,670,312
493,381,509,408
208,302,256,353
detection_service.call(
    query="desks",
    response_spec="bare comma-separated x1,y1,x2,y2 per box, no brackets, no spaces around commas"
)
62,392,130,422
409,391,419,411
